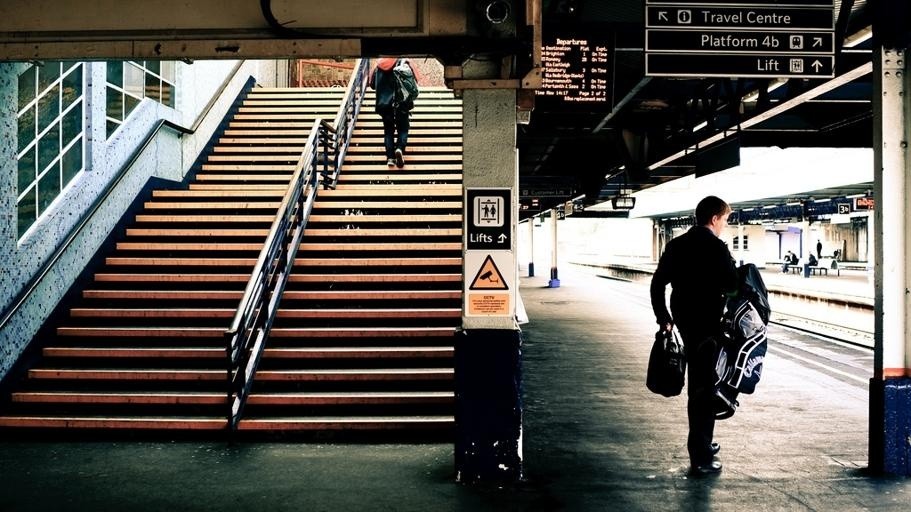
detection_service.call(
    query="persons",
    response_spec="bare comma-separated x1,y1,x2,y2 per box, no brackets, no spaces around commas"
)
369,57,419,168
782,240,822,275
651,196,772,478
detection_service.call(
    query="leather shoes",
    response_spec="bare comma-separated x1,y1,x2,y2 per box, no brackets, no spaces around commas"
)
710,443,720,454
696,461,722,474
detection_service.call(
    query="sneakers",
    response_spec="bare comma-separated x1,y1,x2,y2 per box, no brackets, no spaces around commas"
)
396,149,405,168
387,158,395,166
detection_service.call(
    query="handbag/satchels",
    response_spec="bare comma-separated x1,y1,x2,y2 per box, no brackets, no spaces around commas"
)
714,264,768,416
647,326,686,397
393,63,419,101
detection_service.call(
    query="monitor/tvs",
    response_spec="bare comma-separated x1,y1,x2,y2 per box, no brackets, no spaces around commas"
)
516,19,618,113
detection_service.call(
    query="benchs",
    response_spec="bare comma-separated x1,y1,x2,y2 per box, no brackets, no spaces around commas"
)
808,267,827,276
781,266,802,274
